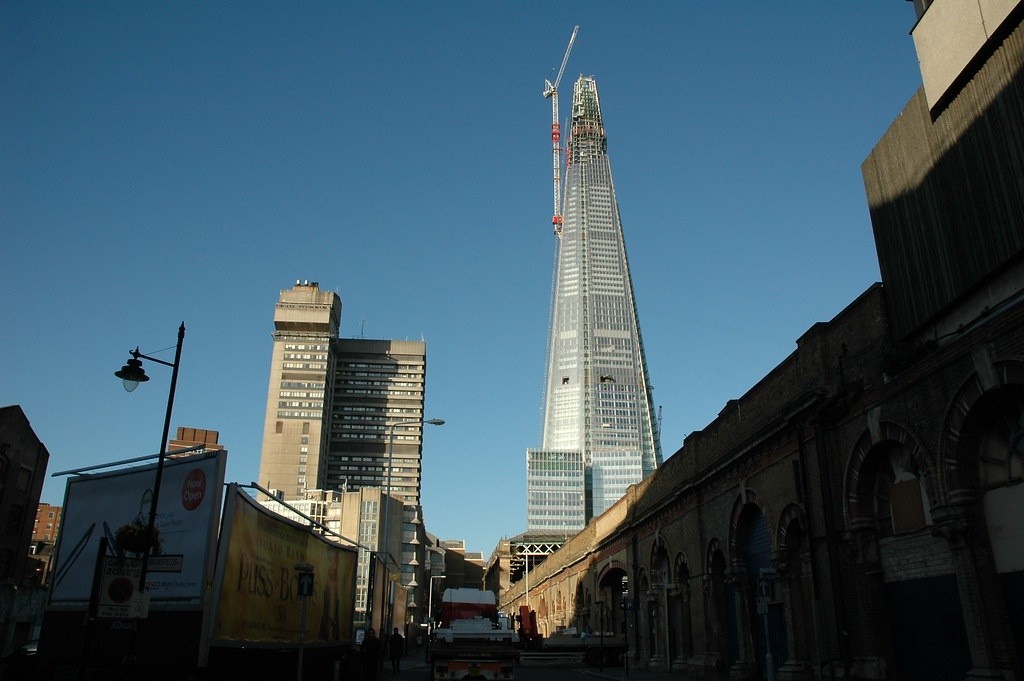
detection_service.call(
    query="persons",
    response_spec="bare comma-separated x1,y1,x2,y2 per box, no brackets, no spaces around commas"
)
351,627,404,681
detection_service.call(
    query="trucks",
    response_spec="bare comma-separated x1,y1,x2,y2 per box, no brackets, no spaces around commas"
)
425,587,520,681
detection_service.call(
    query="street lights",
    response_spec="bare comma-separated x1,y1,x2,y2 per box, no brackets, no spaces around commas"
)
425,575,447,662
381,419,445,671
115,320,186,681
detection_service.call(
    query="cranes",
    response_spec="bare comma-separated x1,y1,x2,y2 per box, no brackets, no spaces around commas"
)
544,24,579,238
